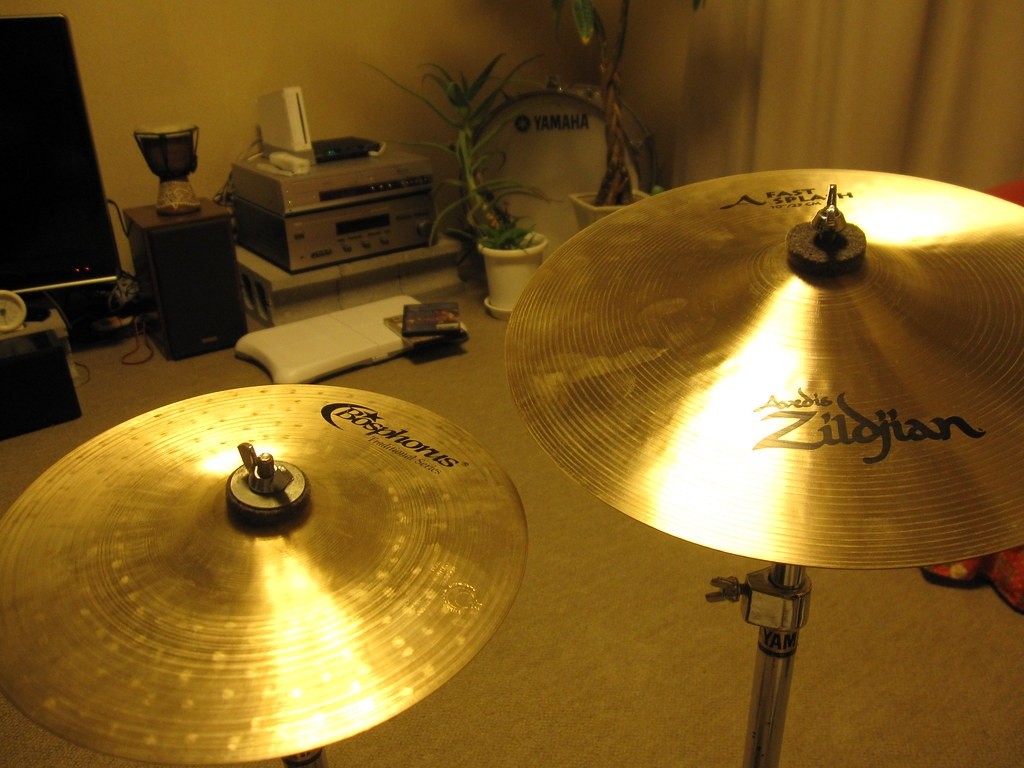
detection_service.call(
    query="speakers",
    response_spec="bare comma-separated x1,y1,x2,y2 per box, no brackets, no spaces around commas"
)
122,196,252,360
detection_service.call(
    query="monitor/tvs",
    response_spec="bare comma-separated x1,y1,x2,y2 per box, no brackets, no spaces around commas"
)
0,15,120,294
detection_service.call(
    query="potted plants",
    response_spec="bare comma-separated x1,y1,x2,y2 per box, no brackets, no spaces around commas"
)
552,0,701,232
367,52,566,320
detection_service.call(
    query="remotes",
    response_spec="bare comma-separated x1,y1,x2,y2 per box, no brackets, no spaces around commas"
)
270,151,310,174
